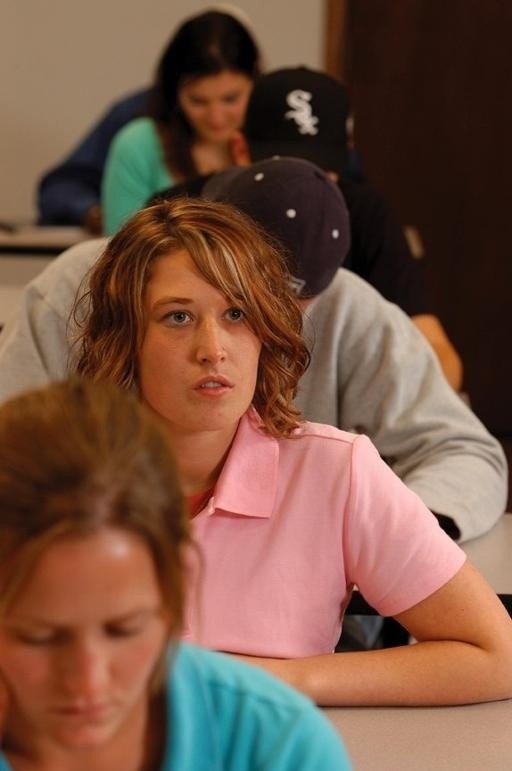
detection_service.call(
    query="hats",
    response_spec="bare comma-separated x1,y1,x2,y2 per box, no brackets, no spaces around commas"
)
245,67,350,167
204,156,350,297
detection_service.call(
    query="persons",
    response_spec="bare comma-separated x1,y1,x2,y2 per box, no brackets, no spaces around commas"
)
144,64,464,393
0,378,351,771
66,195,512,708
99,10,257,238
35,90,155,234
0,156,508,545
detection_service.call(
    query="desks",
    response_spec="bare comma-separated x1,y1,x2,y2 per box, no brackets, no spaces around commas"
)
0,223,95,258
346,508,512,620
314,700,512,770
